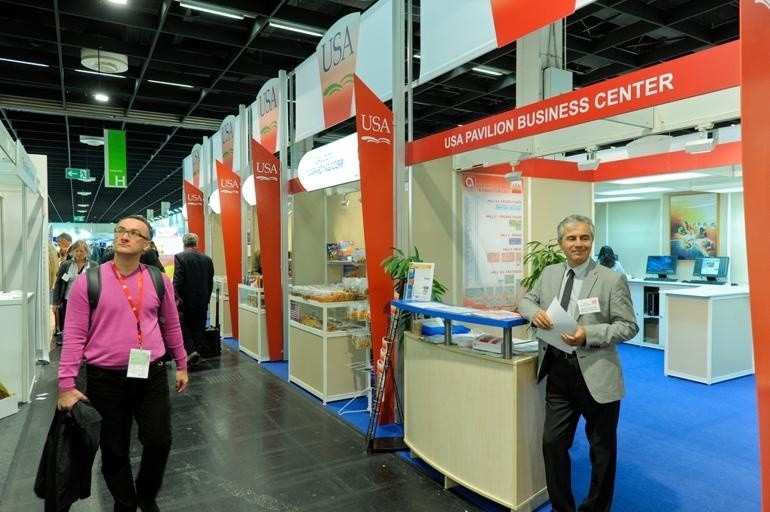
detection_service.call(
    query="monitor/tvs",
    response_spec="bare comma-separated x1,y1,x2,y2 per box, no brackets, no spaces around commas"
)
646,255,678,278
693,256,730,281
594,254,618,262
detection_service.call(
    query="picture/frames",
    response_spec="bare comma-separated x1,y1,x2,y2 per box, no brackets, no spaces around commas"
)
669,192,720,260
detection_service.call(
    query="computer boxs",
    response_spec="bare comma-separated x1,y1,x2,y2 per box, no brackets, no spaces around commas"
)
646,292,659,316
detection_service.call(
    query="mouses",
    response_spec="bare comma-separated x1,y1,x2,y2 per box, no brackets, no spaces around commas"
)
731,282,738,286
681,280,689,283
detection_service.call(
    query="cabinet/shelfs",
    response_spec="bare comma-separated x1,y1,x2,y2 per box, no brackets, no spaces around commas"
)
210,276,233,340
238,283,271,364
288,294,372,406
327,261,368,287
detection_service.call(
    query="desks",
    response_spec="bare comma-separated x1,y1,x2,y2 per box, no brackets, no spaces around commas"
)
622,278,749,350
658,285,755,385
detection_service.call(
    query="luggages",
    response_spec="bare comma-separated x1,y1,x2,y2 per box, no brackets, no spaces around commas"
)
203,289,221,357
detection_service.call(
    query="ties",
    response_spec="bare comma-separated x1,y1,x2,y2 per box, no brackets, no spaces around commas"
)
561,271,575,310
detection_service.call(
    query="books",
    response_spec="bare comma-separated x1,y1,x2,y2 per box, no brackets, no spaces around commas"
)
473,334,505,354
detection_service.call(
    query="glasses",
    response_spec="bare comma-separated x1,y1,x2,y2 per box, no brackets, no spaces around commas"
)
115,227,148,240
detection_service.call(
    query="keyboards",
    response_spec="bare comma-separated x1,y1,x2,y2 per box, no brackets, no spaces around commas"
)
643,277,679,282
688,279,728,285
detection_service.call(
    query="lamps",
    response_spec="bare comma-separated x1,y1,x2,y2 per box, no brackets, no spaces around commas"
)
80,46,128,73
80,135,104,146
577,146,600,172
505,160,522,181
77,178,96,213
340,192,350,207
684,123,717,154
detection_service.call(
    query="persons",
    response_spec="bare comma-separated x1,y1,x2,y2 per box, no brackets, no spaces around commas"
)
595,246,628,279
56,233,165,346
517,216,639,511
173,233,214,367
57,217,189,512
